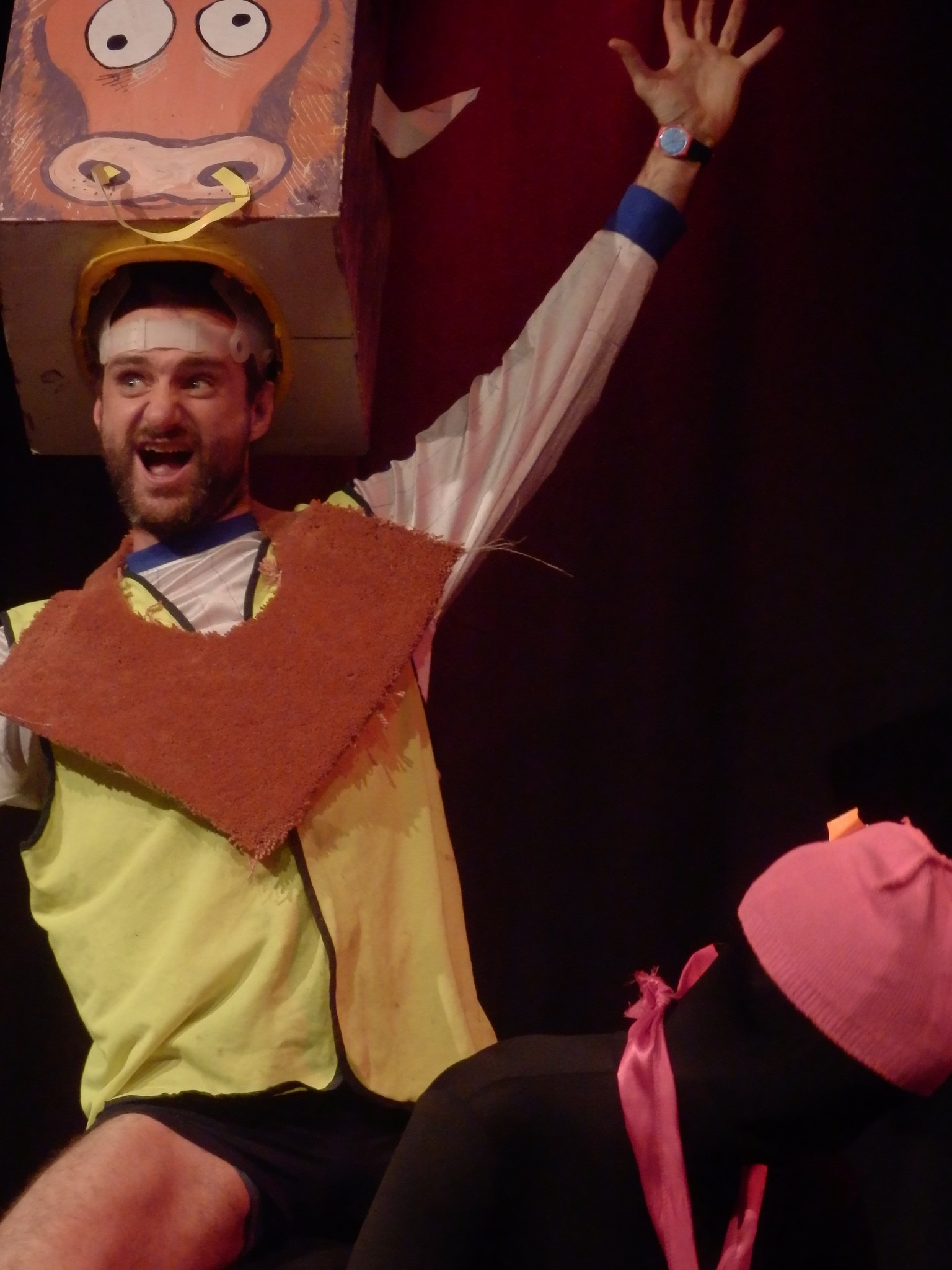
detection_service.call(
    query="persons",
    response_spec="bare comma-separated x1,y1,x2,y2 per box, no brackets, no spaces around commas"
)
355,808,951,1270
0,1,781,1269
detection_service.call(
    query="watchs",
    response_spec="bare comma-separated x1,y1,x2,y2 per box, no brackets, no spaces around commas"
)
653,125,717,166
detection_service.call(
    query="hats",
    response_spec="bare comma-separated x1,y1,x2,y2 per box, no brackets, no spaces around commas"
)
737,805,952,1094
73,232,293,406
